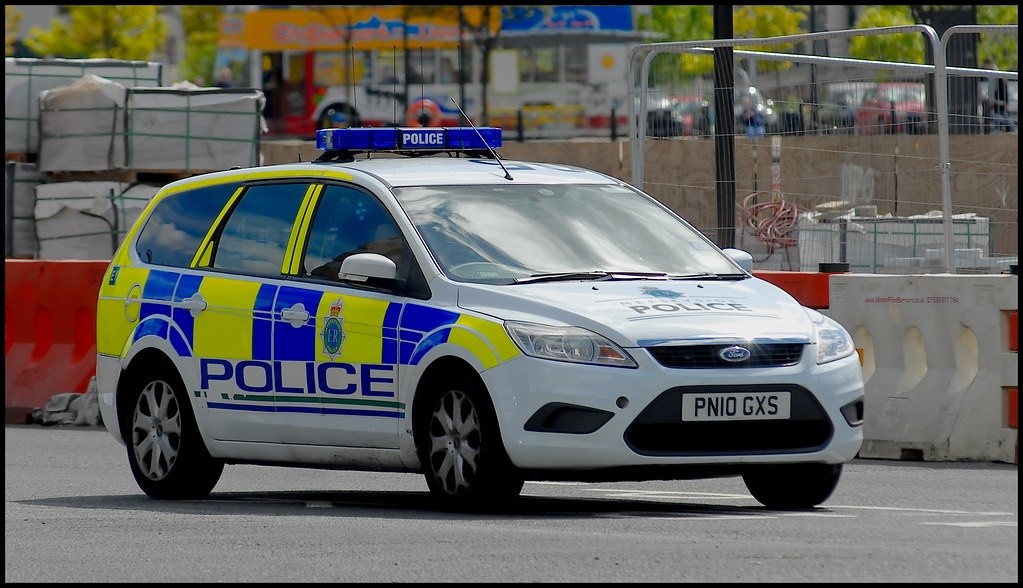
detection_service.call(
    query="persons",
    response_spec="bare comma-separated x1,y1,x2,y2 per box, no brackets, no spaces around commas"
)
523,55,543,81
985,59,1012,128
216,68,232,87
381,58,459,83
742,100,762,136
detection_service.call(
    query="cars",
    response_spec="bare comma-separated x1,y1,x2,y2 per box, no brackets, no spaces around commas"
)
630,75,1018,139
93,123,869,515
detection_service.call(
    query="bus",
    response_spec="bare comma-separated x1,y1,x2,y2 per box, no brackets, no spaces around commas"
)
251,38,638,143
